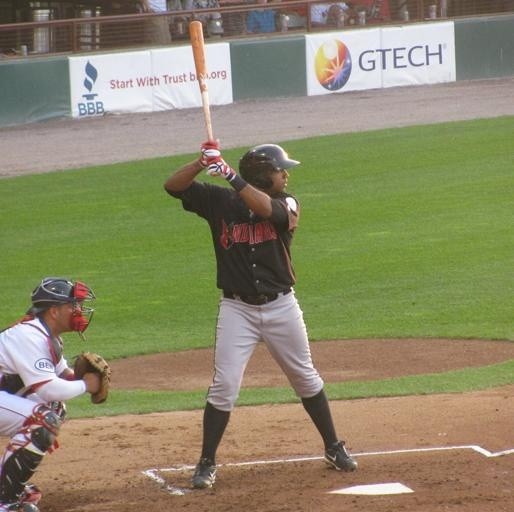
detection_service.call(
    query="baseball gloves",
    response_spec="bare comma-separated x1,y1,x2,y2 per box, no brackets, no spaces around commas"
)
75,351,111,404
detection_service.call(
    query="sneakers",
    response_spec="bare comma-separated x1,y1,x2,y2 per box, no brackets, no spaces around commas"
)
0,485,42,512
325,442,357,471
192,463,216,487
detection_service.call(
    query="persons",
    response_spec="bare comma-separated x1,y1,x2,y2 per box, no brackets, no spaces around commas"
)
164,141,357,486
310,1,448,26
135,1,221,44
245,0,279,34
0,274,112,511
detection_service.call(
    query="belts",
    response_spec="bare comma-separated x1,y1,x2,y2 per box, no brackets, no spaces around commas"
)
223,289,292,305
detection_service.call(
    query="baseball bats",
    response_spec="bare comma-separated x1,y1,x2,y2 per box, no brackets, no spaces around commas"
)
188,21,213,141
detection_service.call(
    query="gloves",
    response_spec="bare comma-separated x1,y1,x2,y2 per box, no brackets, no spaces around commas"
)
199,140,221,168
207,157,236,182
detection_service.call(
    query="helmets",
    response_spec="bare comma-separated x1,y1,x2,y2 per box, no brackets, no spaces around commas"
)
239,144,300,188
26,277,96,341
